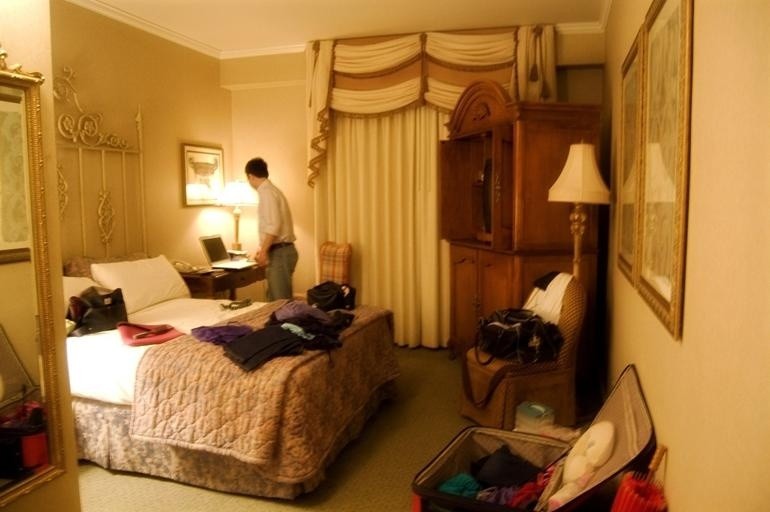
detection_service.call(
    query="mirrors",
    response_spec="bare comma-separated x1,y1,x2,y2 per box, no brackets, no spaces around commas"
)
1,43,67,508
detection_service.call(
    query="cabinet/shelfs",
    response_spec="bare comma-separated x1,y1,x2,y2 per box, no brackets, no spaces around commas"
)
432,77,602,258
447,241,599,367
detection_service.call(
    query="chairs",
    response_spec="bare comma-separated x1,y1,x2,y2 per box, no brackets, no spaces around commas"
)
460,270,588,432
291,240,353,299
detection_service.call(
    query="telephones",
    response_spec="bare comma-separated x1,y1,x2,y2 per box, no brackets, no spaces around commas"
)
170,260,198,273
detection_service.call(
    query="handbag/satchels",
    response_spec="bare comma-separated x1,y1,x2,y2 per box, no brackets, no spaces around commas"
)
65,286,129,336
475,304,548,366
116,319,185,346
306,280,355,312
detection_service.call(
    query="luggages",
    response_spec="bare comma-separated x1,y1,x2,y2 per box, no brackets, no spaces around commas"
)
411,362,658,511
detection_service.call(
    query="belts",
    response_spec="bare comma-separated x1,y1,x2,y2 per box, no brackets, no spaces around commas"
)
269,241,294,252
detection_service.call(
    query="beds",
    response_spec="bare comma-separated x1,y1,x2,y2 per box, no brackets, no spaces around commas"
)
53,63,403,500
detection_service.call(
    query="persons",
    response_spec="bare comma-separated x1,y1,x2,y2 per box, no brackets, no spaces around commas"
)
246,158,298,300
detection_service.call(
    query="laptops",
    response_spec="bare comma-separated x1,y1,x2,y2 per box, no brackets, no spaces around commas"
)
198,234,258,270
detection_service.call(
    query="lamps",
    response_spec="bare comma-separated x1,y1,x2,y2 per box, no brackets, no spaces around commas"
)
219,180,258,253
547,140,611,276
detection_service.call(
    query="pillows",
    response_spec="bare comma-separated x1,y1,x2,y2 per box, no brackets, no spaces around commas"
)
90,252,191,316
62,274,105,333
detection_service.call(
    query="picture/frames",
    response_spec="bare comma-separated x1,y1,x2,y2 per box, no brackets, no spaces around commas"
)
179,139,224,207
641,1,697,341
618,28,639,288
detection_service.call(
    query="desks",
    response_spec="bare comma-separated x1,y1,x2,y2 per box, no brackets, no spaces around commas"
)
179,263,266,300
